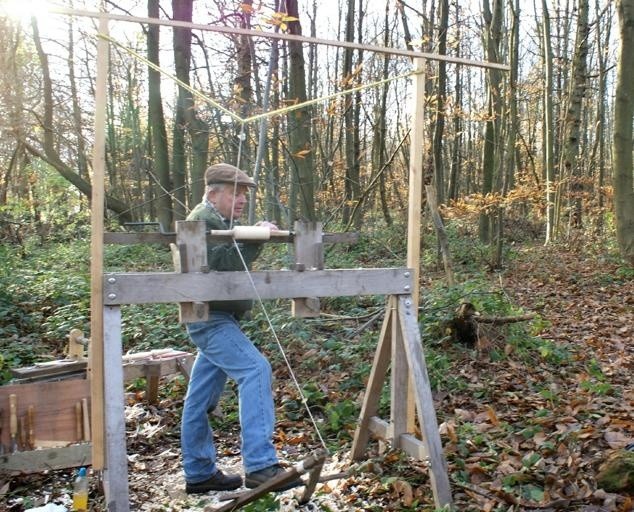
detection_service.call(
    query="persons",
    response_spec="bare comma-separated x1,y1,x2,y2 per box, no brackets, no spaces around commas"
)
177,162,303,495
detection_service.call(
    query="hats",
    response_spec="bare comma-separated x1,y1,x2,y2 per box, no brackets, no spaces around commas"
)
204,163,258,188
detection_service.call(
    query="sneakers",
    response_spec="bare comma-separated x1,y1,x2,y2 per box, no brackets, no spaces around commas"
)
245,463,305,492
185,470,242,493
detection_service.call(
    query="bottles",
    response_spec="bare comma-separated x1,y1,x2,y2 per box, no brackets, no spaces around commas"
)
72,467,90,511
